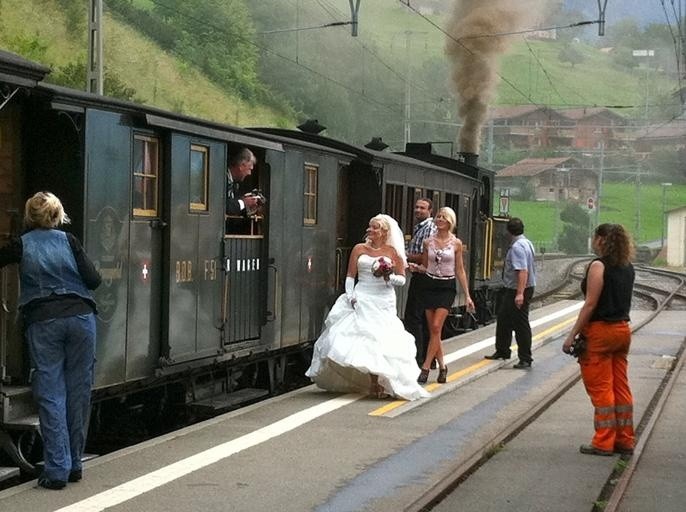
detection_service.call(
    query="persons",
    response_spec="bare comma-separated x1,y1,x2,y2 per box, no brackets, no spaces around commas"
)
484,217,536,368
407,207,475,384
0,190,102,489
405,197,438,370
563,223,635,456
305,214,430,401
226,147,258,215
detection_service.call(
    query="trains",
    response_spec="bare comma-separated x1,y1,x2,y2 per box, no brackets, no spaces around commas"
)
0,46,514,483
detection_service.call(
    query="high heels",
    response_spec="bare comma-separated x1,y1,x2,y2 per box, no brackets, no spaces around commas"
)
438,365,447,383
418,367,429,384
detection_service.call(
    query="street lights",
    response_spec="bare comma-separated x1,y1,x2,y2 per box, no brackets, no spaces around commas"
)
659,181,673,249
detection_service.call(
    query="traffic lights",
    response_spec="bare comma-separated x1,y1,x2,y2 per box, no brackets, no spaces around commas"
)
586,196,595,213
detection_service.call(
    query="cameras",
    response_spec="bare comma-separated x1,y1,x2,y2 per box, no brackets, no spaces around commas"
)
569,333,586,358
244,188,266,216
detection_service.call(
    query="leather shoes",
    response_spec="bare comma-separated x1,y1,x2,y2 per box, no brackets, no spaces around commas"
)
580,444,613,456
484,352,510,359
614,442,633,454
38,471,66,488
69,470,82,482
513,360,531,368
